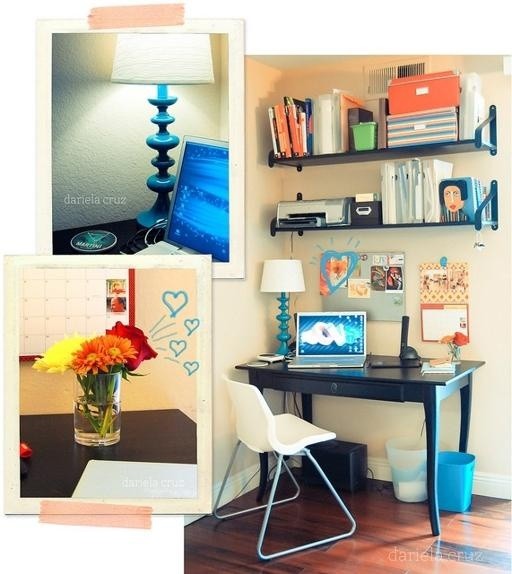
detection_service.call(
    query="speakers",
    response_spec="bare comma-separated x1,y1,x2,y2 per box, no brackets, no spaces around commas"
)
302,440,367,495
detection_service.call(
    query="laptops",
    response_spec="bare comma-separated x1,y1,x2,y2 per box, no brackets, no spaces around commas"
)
288,311,366,369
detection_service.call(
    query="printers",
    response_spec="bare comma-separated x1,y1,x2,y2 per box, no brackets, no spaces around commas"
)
276,197,355,229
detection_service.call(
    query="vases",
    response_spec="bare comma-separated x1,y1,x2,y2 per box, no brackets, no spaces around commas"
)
70,368,123,448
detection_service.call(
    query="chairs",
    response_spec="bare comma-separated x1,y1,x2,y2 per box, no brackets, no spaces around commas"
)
209,374,362,563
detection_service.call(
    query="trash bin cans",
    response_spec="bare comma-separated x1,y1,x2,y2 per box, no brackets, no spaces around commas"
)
436,450,476,513
385,436,428,504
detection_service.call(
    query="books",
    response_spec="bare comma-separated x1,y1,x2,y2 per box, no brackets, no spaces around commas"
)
269,91,373,157
383,158,491,223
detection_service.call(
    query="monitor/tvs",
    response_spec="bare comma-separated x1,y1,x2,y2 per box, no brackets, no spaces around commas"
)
133,136,229,264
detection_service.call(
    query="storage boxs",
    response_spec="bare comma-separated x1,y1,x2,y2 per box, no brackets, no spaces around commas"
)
386,67,462,115
382,107,461,150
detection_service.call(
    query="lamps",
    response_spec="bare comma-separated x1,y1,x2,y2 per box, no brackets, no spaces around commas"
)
259,254,309,357
106,31,219,235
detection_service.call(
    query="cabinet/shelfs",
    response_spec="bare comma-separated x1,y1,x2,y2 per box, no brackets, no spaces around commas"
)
264,101,499,237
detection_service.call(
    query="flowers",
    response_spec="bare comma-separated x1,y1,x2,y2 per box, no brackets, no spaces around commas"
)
27,320,156,392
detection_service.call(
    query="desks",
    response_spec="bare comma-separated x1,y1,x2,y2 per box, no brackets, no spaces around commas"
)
54,207,168,254
234,351,490,547
19,405,196,497
